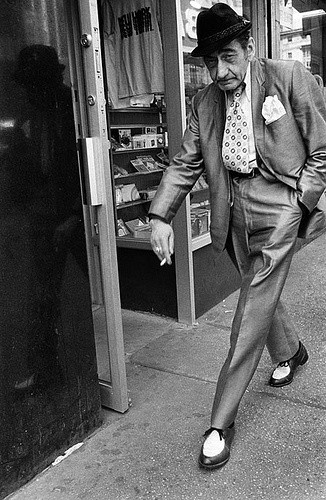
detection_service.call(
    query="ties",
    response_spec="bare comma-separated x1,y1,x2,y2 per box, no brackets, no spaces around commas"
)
222,82,254,174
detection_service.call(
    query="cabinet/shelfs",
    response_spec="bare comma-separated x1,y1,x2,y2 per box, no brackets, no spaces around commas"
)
109,108,221,250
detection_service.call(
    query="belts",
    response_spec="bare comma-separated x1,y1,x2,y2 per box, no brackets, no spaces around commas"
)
228,167,261,180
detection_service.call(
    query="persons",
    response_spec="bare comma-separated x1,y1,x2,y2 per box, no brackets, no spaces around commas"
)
160,253,171,266
147,3,326,469
7,44,89,390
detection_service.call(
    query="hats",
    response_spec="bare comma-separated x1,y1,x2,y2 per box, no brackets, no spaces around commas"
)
190,3,252,58
13,44,67,83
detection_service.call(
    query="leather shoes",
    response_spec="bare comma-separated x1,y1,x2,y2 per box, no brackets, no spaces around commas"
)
196,421,235,469
268,341,308,387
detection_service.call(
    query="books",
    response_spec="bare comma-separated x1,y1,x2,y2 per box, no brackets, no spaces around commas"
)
115,183,141,206
130,159,150,172
119,129,132,150
117,219,131,236
125,219,144,235
136,155,163,172
